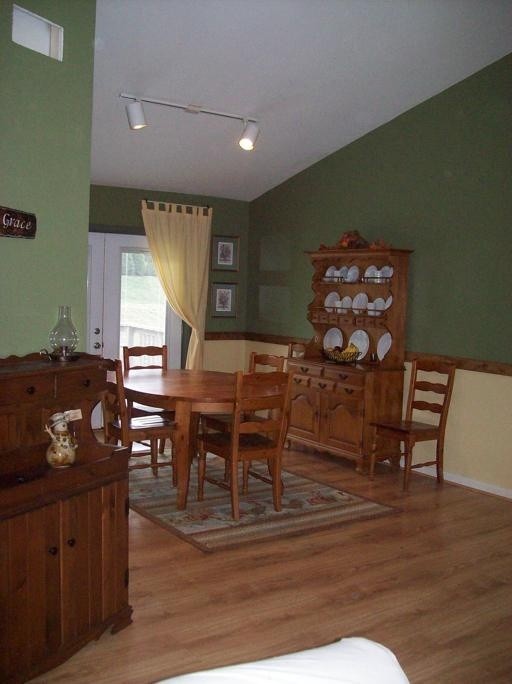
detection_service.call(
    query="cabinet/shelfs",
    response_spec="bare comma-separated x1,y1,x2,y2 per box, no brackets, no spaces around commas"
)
284,230,416,473
0,344,135,684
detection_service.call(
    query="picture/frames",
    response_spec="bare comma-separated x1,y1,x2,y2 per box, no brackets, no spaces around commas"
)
210,280,239,319
210,234,241,271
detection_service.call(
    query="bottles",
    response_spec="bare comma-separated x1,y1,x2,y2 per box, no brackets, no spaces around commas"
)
49,303,79,351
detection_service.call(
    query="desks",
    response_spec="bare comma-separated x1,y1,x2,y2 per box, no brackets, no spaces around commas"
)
105,368,278,512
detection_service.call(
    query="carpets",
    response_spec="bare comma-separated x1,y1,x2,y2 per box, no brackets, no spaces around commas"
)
128,446,404,553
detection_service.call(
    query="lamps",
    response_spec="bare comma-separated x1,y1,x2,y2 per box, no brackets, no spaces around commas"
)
119,91,262,152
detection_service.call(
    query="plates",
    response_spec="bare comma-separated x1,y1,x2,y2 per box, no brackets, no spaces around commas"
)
320,264,394,363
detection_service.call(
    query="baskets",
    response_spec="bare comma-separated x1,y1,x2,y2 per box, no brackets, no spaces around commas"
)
317,347,363,363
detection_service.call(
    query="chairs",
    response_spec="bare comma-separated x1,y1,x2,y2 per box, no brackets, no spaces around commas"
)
266,341,312,449
123,344,170,458
367,357,457,492
100,358,179,489
195,369,294,522
200,351,286,481
